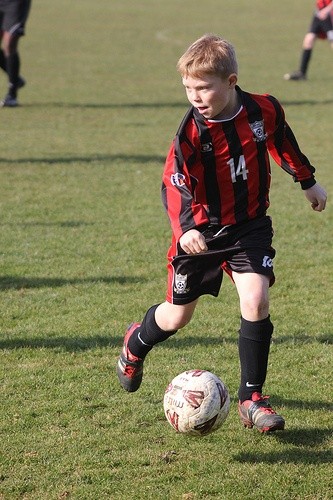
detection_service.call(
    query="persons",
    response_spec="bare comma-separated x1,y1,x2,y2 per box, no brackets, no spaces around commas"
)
0,0,32,107
284,0,333,80
116,34,328,433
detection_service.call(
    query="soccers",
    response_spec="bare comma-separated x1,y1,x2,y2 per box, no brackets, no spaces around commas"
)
162,369,231,438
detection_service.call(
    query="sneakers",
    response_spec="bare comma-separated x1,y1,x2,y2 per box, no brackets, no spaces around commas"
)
3,77,25,106
284,71,306,80
118,323,144,392
239,392,285,432
